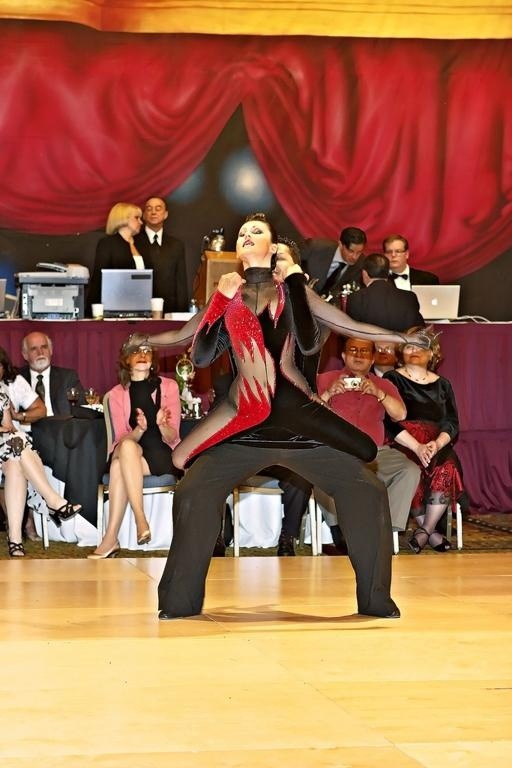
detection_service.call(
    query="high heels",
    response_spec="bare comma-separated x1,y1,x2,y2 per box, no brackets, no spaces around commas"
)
138,523,151,545
48,502,82,527
7,539,25,556
88,542,120,559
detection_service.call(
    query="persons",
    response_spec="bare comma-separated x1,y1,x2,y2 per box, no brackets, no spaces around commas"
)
306,226,367,301
345,254,425,332
313,337,421,554
158,238,400,616
87,332,182,559
85,202,149,318
383,234,440,292
122,213,442,471
379,329,462,554
372,341,404,445
19,331,100,416
0,348,81,558
137,198,193,312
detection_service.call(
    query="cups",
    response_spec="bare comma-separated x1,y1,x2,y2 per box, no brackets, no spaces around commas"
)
150,297,165,317
92,304,104,317
193,403,201,418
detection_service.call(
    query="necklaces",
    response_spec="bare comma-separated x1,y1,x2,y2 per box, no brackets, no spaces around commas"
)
404,366,428,381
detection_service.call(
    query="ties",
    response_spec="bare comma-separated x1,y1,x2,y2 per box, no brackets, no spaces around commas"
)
319,262,347,298
152,234,159,251
35,374,45,401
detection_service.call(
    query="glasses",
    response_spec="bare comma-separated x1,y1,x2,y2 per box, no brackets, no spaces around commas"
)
386,249,406,256
376,345,396,354
342,347,372,355
130,348,151,355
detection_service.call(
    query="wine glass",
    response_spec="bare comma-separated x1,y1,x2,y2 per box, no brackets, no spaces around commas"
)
67,386,81,419
85,389,100,420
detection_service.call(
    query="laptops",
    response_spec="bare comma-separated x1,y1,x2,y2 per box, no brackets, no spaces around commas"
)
412,285,461,319
101,268,153,318
0,278,7,318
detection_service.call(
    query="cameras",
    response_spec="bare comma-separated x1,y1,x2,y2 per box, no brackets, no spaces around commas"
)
344,377,362,389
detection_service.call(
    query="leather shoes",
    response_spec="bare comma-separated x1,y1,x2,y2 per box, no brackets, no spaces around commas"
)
159,612,176,619
388,609,400,619
409,528,430,554
428,529,450,552
277,533,294,556
215,538,226,556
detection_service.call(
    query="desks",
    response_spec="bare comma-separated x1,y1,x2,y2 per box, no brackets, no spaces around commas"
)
0,316,512,517
33,415,334,551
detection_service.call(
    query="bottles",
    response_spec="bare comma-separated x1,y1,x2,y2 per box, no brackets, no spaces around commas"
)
189,298,199,313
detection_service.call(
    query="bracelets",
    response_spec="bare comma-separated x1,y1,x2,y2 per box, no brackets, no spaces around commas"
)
22,412,27,424
379,393,387,402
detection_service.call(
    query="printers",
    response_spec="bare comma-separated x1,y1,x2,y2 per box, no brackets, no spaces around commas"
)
17,262,90,321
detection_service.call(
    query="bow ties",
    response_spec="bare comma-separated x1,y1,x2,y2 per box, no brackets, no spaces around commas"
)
392,273,408,281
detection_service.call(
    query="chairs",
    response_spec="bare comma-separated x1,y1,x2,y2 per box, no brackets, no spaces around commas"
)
41,390,463,556
